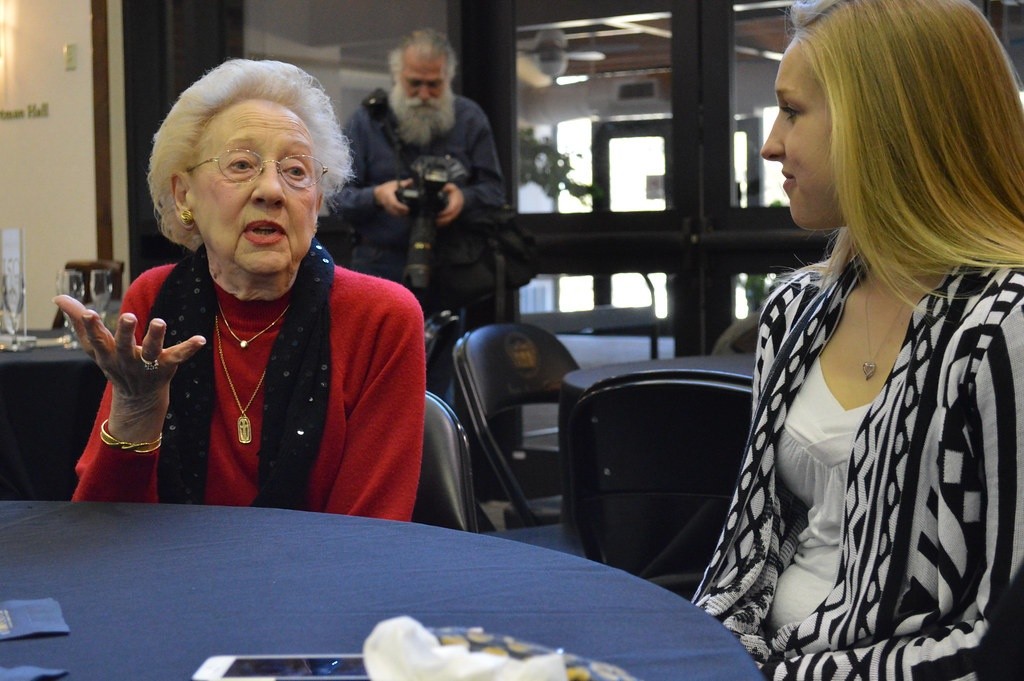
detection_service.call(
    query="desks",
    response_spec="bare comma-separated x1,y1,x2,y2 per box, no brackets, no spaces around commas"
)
0,501,765,681
0,329,116,501
557,353,754,422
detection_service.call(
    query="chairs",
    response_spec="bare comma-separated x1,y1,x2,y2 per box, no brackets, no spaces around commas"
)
558,368,755,603
411,391,477,531
450,323,580,527
707,311,759,355
422,309,463,396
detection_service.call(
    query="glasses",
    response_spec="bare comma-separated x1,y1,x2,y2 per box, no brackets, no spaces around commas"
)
186,148,329,191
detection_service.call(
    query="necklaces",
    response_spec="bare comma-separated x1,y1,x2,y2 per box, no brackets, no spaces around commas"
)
862,289,915,381
215,316,267,444
218,301,289,347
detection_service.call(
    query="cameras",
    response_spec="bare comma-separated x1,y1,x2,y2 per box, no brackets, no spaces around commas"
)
395,189,449,287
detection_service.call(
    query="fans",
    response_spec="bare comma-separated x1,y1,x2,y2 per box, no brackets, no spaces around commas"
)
517,29,606,62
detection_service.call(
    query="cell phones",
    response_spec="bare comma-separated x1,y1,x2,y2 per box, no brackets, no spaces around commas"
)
191,653,371,681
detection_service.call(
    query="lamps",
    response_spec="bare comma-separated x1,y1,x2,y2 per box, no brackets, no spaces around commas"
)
538,59,570,78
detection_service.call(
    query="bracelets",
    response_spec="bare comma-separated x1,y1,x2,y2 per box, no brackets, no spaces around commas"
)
100,419,162,453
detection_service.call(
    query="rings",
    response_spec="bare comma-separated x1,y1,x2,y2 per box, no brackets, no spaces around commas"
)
140,351,158,371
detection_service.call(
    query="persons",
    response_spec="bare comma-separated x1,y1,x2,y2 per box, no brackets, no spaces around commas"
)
52,58,426,521
687,0,1024,681
325,28,505,397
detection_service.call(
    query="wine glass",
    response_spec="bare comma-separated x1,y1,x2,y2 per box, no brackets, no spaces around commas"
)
56,268,112,350
0,227,26,352
